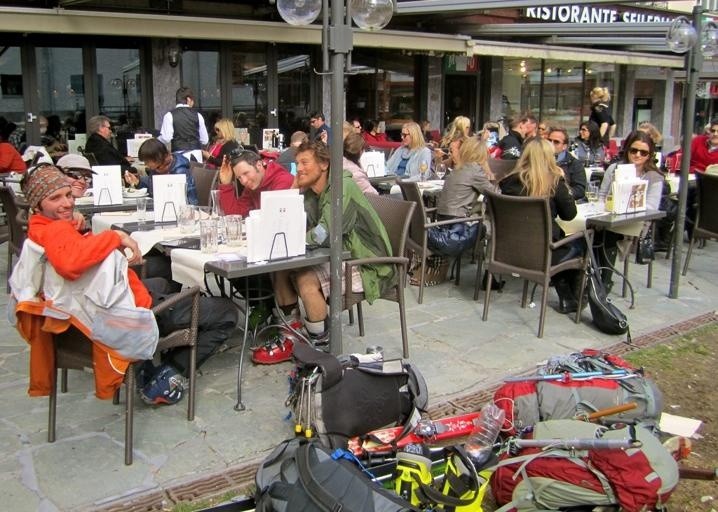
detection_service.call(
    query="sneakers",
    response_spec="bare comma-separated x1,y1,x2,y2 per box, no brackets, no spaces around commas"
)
136,356,187,404
252,323,302,365
291,330,335,361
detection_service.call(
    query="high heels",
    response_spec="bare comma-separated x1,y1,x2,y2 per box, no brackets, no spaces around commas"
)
480,270,505,291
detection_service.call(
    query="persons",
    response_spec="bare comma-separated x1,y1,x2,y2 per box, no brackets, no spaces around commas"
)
1,85,717,406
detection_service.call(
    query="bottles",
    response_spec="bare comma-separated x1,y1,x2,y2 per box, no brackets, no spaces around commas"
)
271,130,278,149
462,402,505,471
675,154,683,178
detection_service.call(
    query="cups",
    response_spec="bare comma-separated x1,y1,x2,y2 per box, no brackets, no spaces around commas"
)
74,185,246,256
572,144,616,214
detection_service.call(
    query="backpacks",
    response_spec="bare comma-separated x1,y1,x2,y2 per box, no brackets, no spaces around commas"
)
497,348,664,438
255,434,424,512
492,427,679,509
286,342,429,435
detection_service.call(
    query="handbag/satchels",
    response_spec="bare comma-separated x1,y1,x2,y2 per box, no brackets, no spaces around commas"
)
589,262,630,334
638,234,660,264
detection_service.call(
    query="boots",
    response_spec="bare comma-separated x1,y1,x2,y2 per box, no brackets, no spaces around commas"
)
554,244,616,313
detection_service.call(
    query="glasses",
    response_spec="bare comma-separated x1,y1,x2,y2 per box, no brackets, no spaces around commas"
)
548,137,566,146
399,132,410,138
58,167,94,182
629,146,652,159
581,128,588,131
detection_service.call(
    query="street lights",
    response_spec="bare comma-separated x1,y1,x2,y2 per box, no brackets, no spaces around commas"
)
246,77,267,117
112,72,138,126
662,4,718,300
277,0,395,363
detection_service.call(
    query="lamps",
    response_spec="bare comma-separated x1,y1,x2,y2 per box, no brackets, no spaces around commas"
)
168,48,180,68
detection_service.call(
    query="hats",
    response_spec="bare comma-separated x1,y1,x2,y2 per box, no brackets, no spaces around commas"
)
56,155,99,175
19,162,66,209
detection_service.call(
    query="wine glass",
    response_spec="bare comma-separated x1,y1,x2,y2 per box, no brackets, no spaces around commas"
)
416,158,447,190
665,157,674,179
